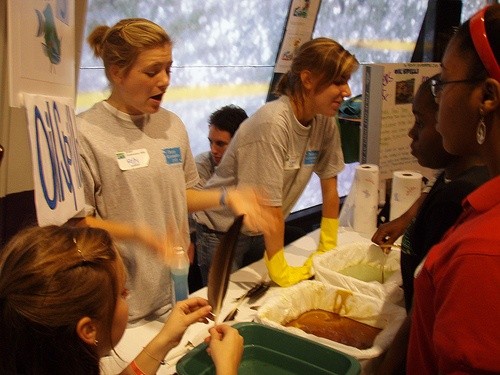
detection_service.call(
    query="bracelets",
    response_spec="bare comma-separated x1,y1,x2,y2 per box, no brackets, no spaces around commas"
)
219,190,226,206
143,346,165,365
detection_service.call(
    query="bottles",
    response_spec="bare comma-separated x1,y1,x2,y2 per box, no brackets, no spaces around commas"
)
170,246,190,305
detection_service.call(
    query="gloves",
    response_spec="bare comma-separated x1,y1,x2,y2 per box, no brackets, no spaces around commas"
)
305,217,339,265
264,248,314,287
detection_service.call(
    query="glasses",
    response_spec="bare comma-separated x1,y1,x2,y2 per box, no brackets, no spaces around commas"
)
430,78,487,96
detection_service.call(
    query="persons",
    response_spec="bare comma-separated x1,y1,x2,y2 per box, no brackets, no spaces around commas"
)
406,3,500,375
401,72,491,315
67,19,276,329
196,37,360,290
372,168,445,255
193,103,248,188
0,225,245,375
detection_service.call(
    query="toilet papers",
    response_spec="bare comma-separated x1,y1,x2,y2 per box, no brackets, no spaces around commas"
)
388,171,423,222
338,164,379,234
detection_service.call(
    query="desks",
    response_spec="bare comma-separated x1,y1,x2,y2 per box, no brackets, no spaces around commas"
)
99,226,404,375
